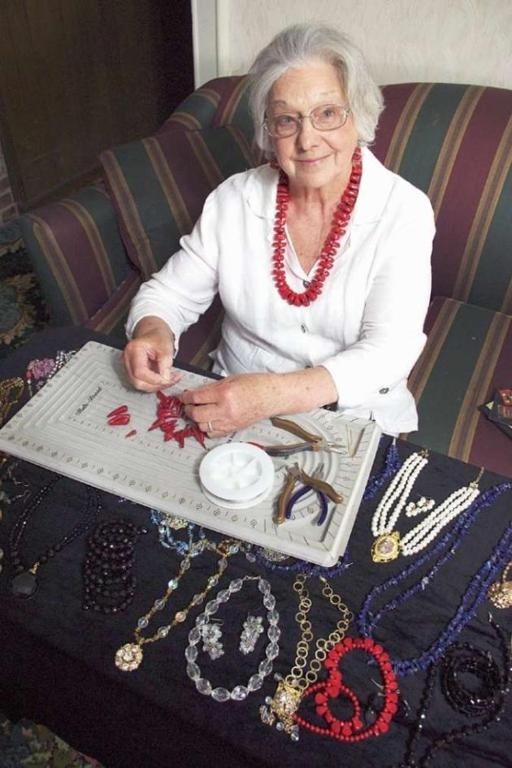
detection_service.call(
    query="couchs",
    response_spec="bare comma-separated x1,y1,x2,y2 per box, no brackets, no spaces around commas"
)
14,66,512,485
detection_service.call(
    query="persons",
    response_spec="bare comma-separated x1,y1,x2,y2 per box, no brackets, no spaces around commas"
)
123,20,438,446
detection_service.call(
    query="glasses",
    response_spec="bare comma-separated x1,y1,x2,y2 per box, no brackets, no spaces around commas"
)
260,101,351,140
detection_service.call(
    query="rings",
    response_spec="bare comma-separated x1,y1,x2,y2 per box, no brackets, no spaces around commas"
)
207,420,214,435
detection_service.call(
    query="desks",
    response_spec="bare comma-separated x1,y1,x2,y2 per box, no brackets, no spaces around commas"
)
1,321,510,767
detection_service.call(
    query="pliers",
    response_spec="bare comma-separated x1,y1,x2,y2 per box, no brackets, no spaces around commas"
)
265,416,348,526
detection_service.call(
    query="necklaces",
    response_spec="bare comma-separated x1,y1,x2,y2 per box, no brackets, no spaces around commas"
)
273,145,364,307
109,439,512,768
0,349,102,600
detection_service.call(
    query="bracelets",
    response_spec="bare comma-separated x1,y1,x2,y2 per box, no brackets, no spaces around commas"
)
80,518,144,615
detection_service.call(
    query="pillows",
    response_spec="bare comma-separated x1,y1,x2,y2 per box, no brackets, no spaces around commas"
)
92,123,264,280
